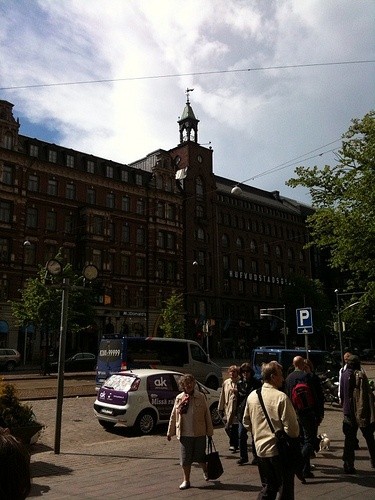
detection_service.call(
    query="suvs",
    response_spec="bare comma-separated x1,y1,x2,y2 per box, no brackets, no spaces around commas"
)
0,349,21,371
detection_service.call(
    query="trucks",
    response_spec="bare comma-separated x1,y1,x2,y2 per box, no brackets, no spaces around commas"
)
94,335,223,392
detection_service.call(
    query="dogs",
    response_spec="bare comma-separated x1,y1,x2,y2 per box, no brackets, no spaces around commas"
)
318,432,331,452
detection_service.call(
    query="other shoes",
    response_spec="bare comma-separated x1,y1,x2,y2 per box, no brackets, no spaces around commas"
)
229,446,236,452
344,467,357,474
179,481,191,490
353,443,361,450
236,458,249,465
252,458,258,465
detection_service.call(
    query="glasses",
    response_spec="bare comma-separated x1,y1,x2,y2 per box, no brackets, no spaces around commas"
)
243,370,249,373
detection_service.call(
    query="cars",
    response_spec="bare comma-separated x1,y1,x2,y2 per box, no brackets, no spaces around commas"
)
328,346,375,362
42,352,96,373
91,368,226,437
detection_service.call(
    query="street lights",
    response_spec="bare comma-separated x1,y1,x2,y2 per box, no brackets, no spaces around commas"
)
334,289,369,364
39,257,98,454
258,305,287,350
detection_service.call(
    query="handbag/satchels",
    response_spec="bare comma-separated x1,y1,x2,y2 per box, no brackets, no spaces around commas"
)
275,431,309,475
355,371,375,428
198,436,224,481
290,383,314,413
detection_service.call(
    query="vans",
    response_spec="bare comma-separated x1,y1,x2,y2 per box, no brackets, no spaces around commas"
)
251,349,342,386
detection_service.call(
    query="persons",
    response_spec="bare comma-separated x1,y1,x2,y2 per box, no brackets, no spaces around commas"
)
304,363,325,435
237,362,263,465
243,361,299,500
285,356,315,484
217,365,242,452
166,374,213,490
341,355,361,473
338,352,375,468
